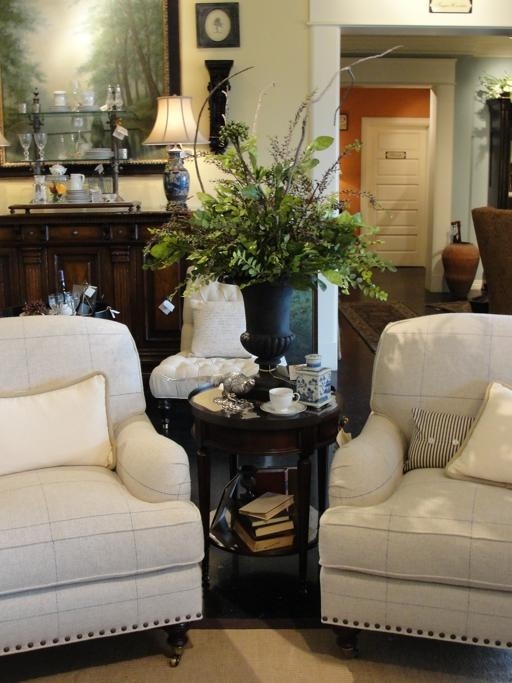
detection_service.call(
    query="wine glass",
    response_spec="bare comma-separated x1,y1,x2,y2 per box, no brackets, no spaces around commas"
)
48,294,63,315
35,133,49,162
17,132,32,161
70,128,80,160
66,292,80,317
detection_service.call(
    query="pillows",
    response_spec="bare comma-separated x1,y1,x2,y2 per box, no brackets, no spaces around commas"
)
1,372,118,475
446,381,512,490
403,407,476,474
190,299,253,357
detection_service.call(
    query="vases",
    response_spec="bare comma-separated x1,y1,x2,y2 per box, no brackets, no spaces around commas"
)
442,242,481,298
476,72,512,99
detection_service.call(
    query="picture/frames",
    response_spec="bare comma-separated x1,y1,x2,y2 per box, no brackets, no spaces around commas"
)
341,114,349,130
197,2,240,50
1,1,181,176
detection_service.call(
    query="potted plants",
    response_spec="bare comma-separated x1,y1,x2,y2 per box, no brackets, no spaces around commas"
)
142,42,405,403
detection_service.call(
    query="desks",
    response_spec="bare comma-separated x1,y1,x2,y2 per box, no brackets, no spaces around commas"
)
187,379,346,590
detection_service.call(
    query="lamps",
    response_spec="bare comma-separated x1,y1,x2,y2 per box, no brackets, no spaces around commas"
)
142,96,212,211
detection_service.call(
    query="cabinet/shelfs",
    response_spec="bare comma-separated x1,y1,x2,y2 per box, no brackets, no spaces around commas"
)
0,211,188,384
485,96,511,210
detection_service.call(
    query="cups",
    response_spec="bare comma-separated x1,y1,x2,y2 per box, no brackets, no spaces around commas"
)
89,184,102,202
17,103,27,114
118,148,128,160
269,387,300,412
69,173,85,190
52,91,68,106
304,353,322,370
102,193,117,202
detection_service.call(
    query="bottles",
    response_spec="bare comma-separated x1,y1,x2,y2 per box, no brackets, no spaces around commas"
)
75,283,93,316
57,270,76,315
115,83,123,109
32,175,47,204
106,84,114,111
58,134,68,160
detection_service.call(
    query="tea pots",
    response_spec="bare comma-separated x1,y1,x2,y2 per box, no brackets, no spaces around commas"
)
49,162,67,176
79,91,94,106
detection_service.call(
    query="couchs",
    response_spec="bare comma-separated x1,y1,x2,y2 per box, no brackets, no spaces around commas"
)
149,282,247,438
0,315,205,668
317,313,512,662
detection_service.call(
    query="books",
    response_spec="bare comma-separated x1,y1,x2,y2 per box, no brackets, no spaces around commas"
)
251,520,294,538
231,519,295,553
239,492,294,520
247,515,289,527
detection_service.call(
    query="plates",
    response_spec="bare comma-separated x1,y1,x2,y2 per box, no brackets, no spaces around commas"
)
86,148,113,159
66,189,92,204
259,402,308,417
48,105,72,113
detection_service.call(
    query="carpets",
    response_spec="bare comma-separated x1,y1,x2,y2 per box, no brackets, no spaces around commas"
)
339,297,418,354
